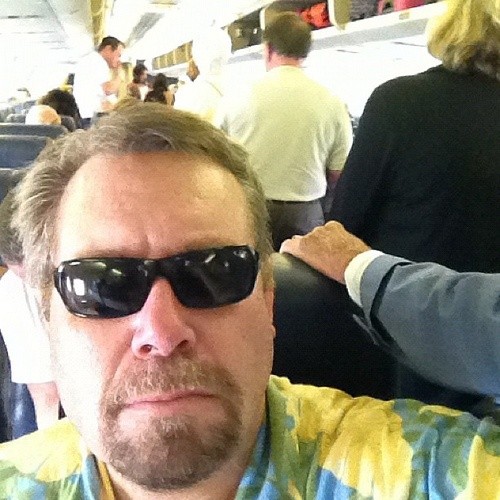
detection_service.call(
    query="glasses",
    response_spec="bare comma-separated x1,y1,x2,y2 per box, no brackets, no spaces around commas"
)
45,246,269,319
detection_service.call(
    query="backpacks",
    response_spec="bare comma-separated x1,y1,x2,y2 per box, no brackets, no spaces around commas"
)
228,21,252,50
288,0,330,30
339,0,384,20
391,0,438,10
251,15,264,45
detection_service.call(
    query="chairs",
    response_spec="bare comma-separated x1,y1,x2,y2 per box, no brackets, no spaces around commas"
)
0,100,90,442
269,254,392,401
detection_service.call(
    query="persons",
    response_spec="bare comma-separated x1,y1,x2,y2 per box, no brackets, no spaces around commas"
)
280,218,500,397
221,12,352,248
1,189,60,439
22,30,234,128
0,103,500,500
321,0,500,270
